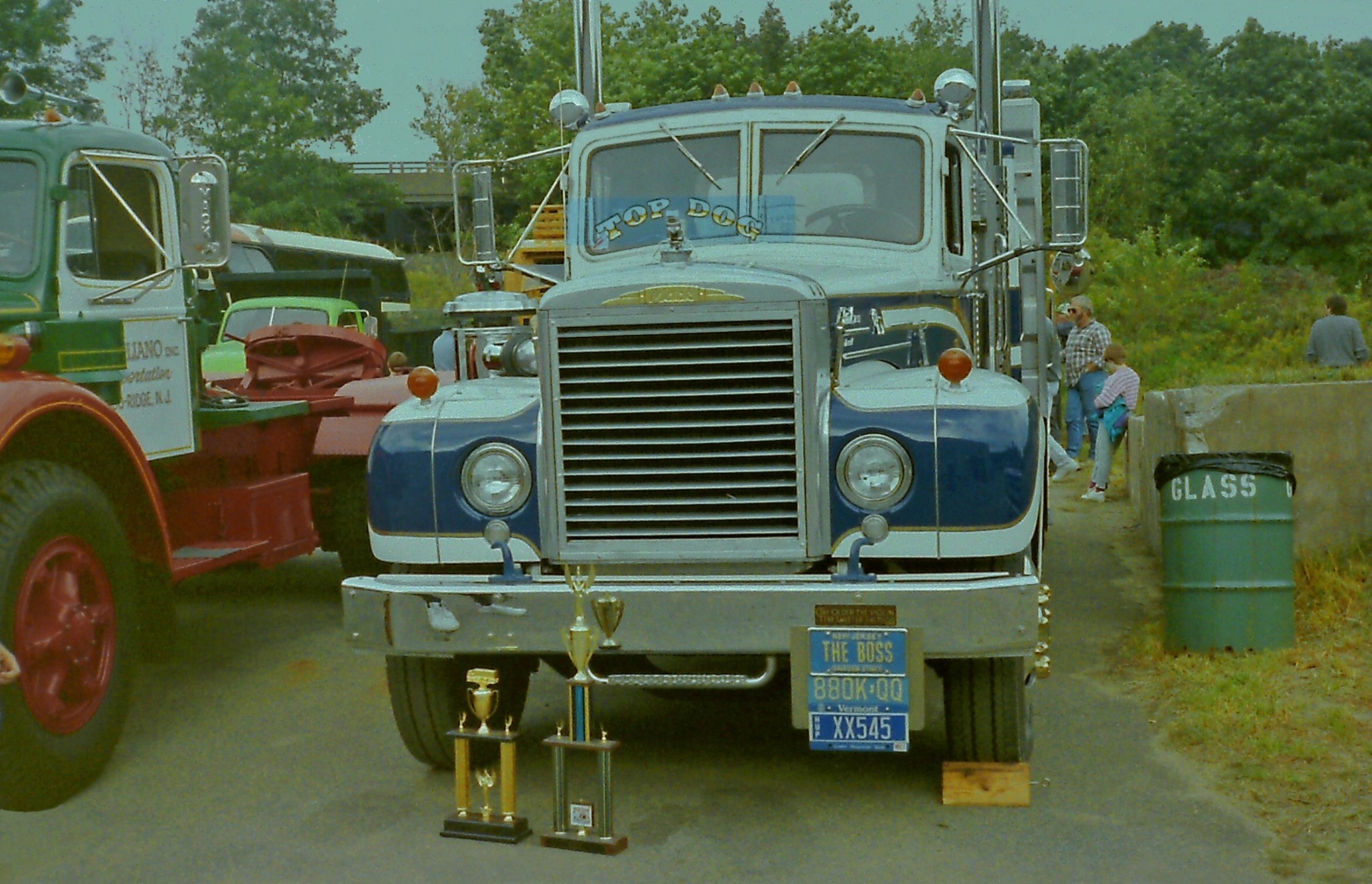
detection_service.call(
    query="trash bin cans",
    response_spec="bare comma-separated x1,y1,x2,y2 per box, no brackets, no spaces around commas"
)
1157,451,1296,656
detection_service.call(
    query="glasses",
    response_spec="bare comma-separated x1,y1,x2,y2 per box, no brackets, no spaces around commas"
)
1067,308,1078,314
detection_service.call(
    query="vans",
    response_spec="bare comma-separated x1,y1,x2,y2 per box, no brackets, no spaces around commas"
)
65,209,413,337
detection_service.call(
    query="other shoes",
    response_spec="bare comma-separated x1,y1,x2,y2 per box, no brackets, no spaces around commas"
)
1081,487,1105,503
1052,460,1081,482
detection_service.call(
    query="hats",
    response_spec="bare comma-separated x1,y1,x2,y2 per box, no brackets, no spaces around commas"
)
1056,302,1073,318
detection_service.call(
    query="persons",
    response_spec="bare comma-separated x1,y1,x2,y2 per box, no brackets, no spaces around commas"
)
1305,295,1370,367
1081,342,1141,504
1045,317,1078,482
1066,297,1112,471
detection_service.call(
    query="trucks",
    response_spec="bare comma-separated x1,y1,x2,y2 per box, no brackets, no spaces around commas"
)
339,0,1093,770
1,70,455,811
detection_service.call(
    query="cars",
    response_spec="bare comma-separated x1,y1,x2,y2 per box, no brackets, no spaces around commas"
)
201,297,379,384
